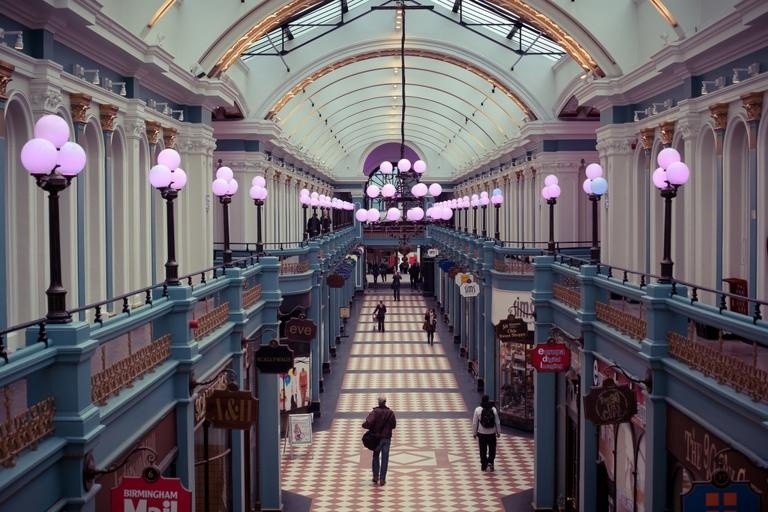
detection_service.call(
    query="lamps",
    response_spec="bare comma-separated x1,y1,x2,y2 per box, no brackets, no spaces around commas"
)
148,148,187,298
212,167,238,268
583,162,608,265
541,174,562,252
21,114,86,324
651,147,688,285
249,176,267,253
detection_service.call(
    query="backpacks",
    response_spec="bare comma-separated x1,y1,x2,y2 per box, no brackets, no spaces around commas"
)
481,407,496,428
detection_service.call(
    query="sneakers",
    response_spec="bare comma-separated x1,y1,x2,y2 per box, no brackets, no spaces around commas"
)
380,478,386,485
372,474,379,483
488,462,495,472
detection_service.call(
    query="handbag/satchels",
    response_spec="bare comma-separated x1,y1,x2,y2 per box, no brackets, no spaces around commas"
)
361,429,381,450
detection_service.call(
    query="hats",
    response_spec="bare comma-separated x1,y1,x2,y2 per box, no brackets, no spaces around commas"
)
377,394,389,403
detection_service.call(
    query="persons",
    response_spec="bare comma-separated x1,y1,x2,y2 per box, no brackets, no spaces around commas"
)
425,307,438,345
389,262,420,289
362,397,396,485
320,210,332,233
374,300,386,332
280,374,288,411
472,393,501,473
379,262,386,281
308,213,320,238
299,368,308,406
367,256,372,274
290,367,298,408
372,261,380,284
391,272,402,301
